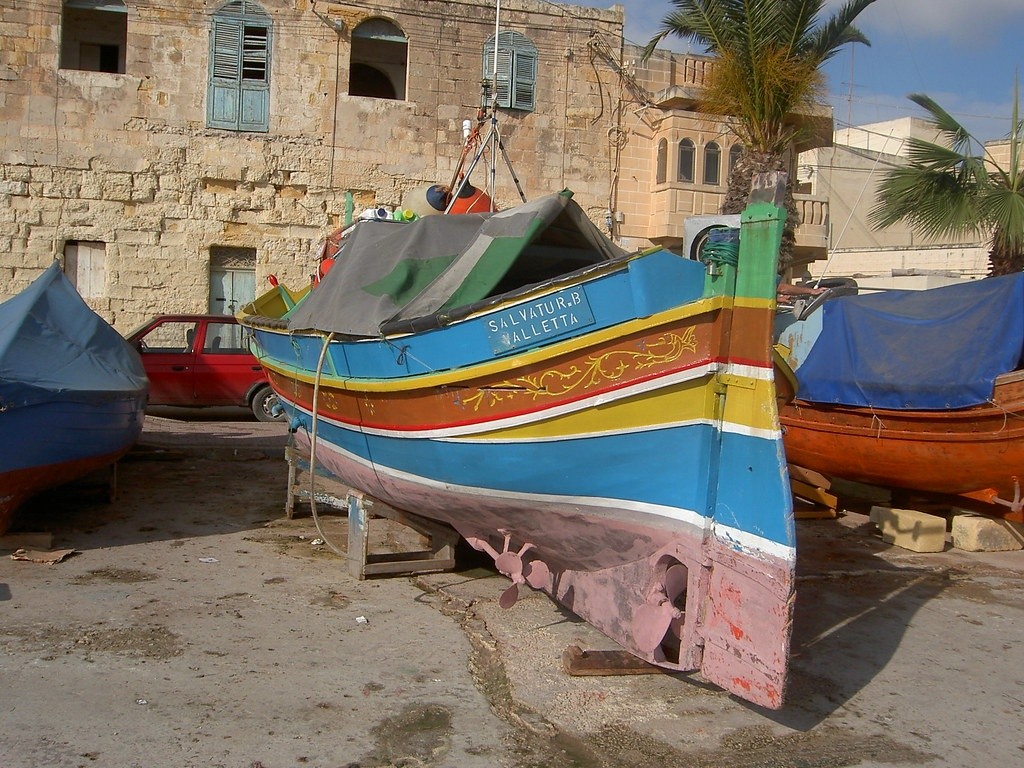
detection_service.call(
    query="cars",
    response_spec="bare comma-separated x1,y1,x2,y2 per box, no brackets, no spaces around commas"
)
121,313,292,423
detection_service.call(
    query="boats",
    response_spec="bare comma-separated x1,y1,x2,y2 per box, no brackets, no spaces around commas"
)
232,0,798,710
770,272,1023,509
0,258,152,538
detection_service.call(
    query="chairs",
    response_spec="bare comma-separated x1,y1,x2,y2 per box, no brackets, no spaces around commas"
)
183,329,194,353
211,336,222,353
